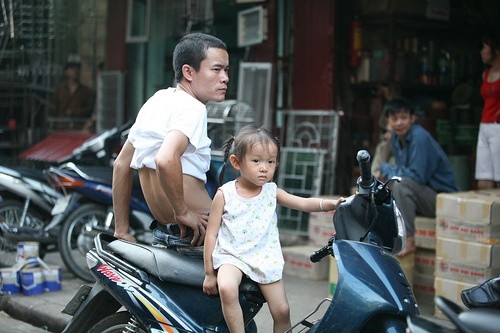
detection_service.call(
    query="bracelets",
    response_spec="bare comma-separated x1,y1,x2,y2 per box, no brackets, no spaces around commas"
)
319,197,328,212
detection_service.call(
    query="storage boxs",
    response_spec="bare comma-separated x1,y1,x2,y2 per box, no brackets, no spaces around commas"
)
328,252,414,300
282,196,346,281
415,190,500,319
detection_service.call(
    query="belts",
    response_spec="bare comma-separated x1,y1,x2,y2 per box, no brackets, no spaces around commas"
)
149,220,191,235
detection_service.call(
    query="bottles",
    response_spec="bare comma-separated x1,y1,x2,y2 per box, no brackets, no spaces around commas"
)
393,37,466,88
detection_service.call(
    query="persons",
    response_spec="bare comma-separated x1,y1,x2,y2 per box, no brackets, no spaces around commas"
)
38,55,97,141
476,33,500,190
112,32,230,256
373,98,462,258
203,124,346,333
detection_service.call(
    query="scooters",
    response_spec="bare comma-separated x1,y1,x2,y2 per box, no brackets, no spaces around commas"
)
433,294,500,333
0,118,137,269
41,102,240,284
56,148,458,333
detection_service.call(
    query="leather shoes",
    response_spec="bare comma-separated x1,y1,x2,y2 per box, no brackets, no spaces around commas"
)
462,278,499,309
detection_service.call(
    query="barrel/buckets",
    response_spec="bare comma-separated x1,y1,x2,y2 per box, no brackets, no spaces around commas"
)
17,241,39,268
42,266,63,292
0,268,21,294
20,268,44,295
